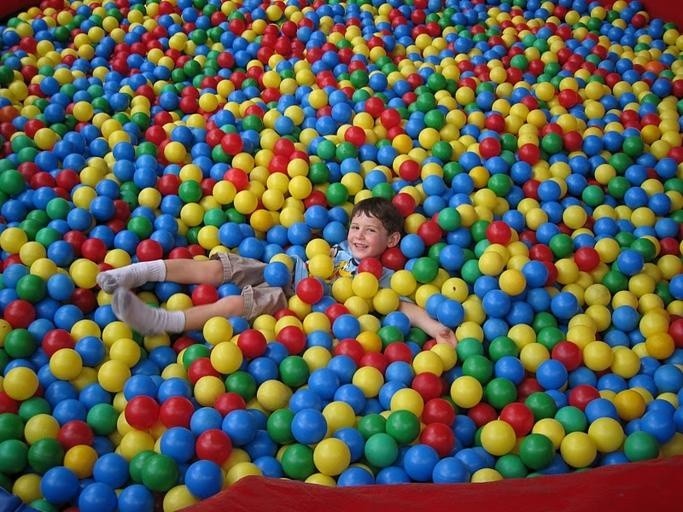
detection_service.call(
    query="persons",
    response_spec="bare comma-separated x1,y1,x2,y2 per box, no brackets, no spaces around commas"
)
96,196,458,348
